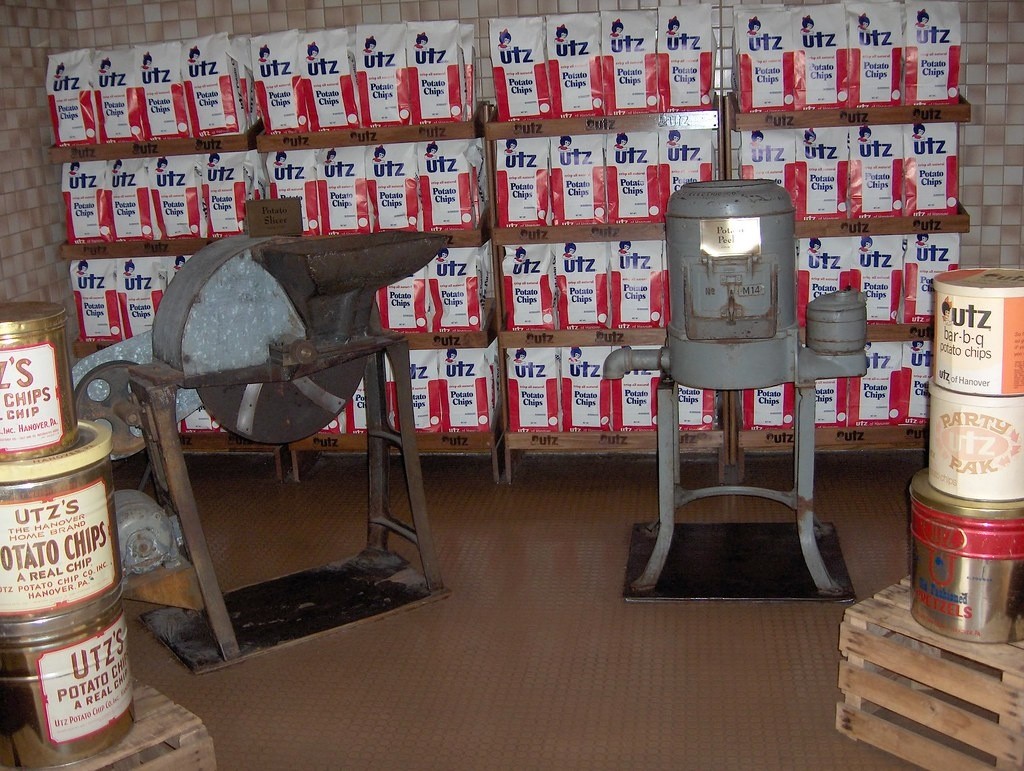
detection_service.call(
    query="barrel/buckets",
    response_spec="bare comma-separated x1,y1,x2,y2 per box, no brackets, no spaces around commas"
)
0,301,135,768
909,267,1024,643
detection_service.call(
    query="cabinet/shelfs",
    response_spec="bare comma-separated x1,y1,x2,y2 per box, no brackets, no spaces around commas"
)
482,93,731,483
48,117,291,480
725,86,972,484
257,103,507,485
